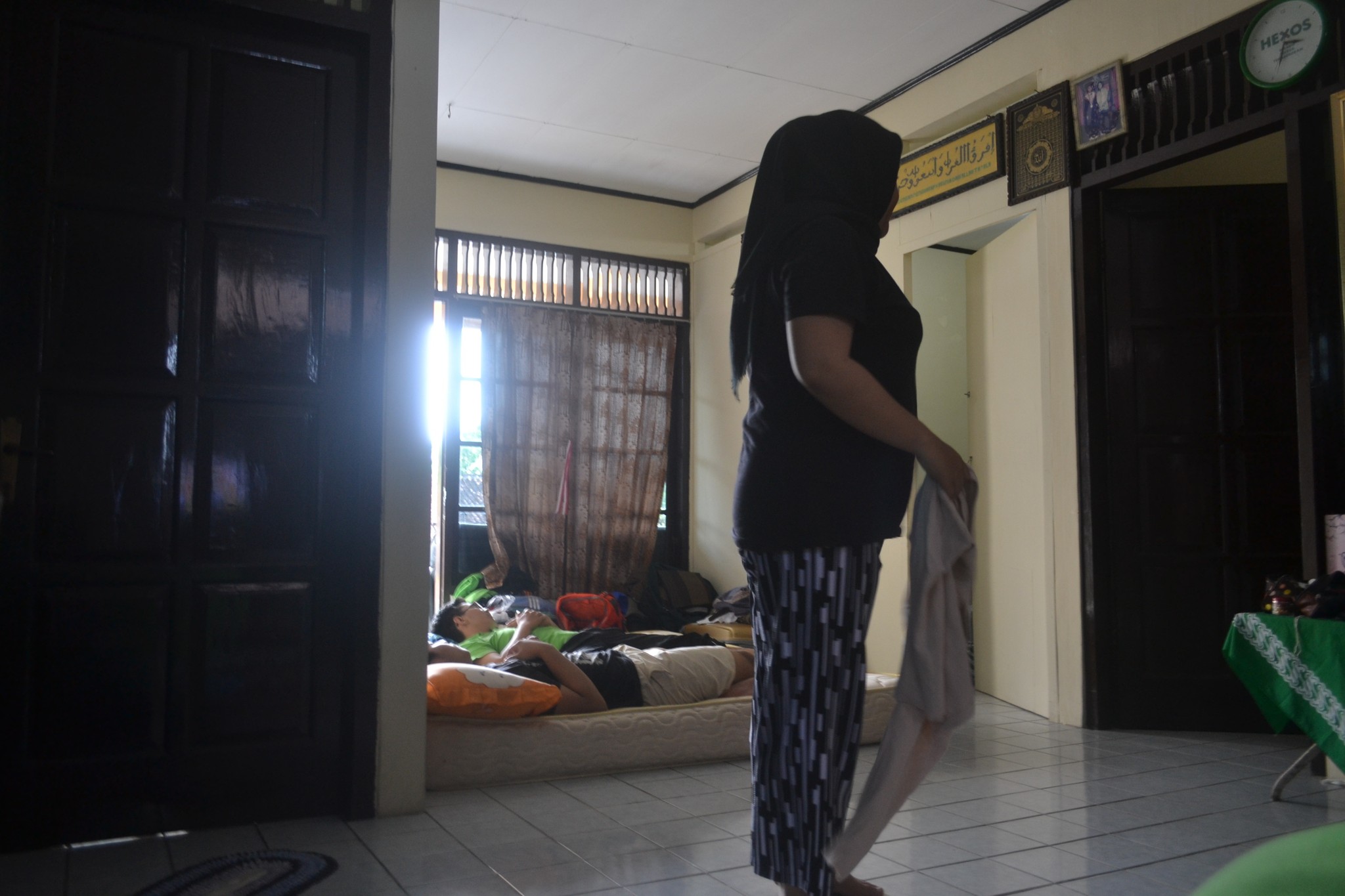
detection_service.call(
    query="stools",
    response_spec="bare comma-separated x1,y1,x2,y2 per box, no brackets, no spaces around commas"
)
682,620,754,648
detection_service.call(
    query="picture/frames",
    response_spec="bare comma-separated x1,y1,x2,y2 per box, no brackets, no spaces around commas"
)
1069,57,1127,151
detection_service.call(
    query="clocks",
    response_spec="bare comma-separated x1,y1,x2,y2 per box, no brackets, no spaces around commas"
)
1239,0,1329,90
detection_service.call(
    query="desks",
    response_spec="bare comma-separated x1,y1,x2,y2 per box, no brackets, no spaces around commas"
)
1221,613,1344,801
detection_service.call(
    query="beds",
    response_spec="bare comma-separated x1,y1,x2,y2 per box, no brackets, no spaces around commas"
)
428,673,903,790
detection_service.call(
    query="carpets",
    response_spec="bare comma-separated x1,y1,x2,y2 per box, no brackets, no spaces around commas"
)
126,846,340,896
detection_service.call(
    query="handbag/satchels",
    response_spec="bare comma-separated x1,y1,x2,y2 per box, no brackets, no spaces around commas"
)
555,590,628,633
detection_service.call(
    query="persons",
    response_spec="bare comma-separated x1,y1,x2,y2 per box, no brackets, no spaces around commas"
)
1082,81,1099,140
427,635,756,717
1096,82,1111,136
730,108,969,896
429,598,727,668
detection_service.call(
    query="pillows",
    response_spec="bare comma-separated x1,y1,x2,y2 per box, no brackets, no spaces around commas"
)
427,660,563,719
